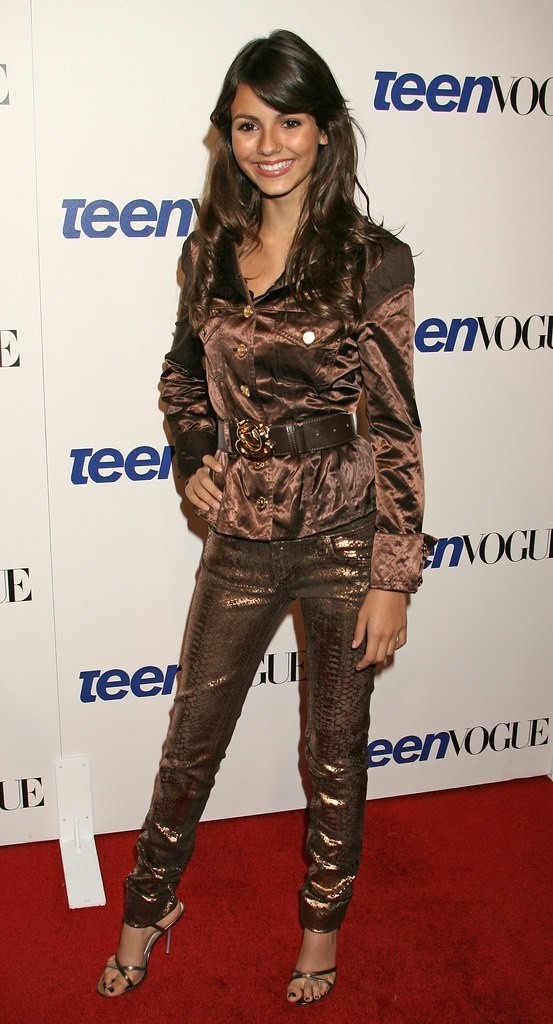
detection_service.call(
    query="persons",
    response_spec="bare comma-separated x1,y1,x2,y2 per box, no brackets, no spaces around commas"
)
98,28,439,1005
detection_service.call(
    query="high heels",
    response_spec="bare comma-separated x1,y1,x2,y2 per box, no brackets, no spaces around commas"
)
97,901,184,998
287,925,338,1006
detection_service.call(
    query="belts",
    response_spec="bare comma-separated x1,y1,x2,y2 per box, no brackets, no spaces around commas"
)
216,411,357,460
